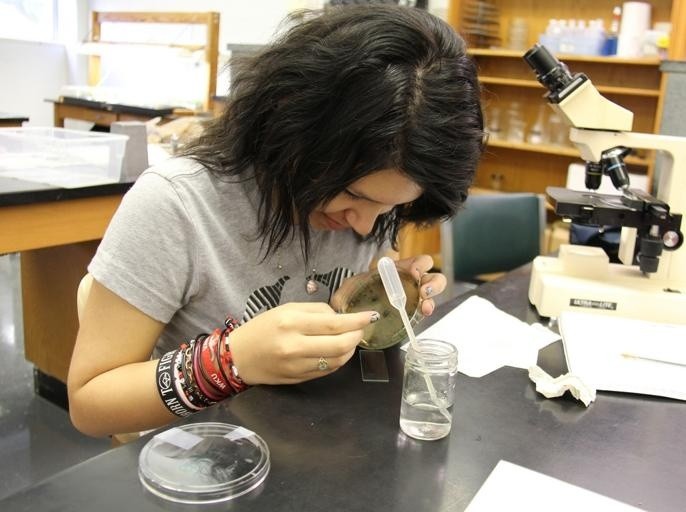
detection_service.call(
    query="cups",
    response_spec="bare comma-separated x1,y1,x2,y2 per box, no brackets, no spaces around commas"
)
397,338,460,442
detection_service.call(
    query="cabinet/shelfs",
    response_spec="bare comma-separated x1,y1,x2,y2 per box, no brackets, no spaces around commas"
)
399,2,686,260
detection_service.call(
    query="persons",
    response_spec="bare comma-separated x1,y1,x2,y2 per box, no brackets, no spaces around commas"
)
64,3,491,439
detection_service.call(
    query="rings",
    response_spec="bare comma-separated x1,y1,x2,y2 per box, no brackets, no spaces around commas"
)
319,354,328,372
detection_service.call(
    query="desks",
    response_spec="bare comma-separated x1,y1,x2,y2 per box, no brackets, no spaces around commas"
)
0,95,212,412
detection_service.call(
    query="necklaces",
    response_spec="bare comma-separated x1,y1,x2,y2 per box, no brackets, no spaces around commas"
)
267,217,324,295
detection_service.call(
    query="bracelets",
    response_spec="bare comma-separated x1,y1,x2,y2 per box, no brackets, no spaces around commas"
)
155,315,251,420
